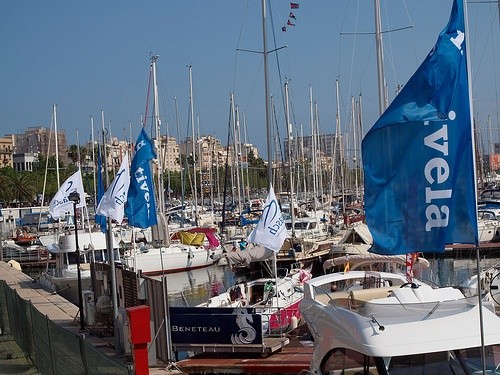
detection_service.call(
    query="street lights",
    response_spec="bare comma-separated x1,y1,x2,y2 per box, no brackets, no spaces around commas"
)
67,190,87,332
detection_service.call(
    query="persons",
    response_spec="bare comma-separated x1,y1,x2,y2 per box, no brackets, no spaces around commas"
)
239,238,247,251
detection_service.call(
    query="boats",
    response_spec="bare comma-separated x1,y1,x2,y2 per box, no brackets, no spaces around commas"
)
298,269,500,375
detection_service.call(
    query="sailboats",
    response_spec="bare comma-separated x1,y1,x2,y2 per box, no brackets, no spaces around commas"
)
8,0,500,336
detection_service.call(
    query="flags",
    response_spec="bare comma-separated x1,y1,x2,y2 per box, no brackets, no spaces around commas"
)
125,127,157,229
95,148,130,224
94,144,107,234
244,182,291,253
360,0,478,256
49,170,86,220
282,2,299,32
